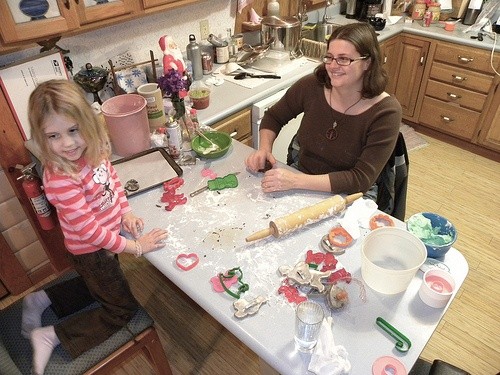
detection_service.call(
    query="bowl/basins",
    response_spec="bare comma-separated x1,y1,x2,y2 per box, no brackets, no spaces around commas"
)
191,131,232,159
406,213,457,258
368,16,385,29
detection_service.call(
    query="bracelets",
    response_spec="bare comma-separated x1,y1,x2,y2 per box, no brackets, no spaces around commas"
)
134,239,143,258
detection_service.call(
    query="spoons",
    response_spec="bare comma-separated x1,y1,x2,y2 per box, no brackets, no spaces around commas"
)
236,71,282,80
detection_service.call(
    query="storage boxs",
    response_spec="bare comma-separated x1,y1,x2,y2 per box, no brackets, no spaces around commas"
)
0,49,72,141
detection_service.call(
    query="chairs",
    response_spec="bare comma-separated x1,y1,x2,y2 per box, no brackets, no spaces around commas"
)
286,130,409,222
0,269,173,375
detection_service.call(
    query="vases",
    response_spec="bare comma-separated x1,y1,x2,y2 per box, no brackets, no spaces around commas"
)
167,99,196,151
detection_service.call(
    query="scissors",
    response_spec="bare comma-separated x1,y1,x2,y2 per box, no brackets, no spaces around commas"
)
470,33,484,41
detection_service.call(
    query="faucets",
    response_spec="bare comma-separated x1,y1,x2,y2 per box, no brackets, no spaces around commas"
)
296,1,309,23
322,0,331,24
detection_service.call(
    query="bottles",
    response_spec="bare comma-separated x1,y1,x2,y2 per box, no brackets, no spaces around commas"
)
187,33,203,80
382,0,441,23
266,0,280,16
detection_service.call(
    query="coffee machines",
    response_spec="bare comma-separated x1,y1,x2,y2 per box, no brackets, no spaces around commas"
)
346,0,383,20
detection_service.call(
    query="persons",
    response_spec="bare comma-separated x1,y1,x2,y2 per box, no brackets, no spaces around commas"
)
21,79,167,375
244,20,403,211
158,35,188,98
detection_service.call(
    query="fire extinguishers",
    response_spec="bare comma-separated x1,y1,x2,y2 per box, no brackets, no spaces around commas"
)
15,161,57,232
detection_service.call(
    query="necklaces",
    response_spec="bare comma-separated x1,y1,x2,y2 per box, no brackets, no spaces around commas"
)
326,90,363,141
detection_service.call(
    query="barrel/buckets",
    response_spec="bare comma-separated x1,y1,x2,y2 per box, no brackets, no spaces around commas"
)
100,93,150,157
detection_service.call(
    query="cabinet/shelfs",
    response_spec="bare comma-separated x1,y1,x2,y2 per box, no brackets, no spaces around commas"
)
0,0,201,55
211,105,255,150
378,32,500,163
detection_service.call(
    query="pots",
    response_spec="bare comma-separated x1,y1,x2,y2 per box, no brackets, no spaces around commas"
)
260,16,302,59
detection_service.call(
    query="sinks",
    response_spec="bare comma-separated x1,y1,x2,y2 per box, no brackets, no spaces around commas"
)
301,23,342,43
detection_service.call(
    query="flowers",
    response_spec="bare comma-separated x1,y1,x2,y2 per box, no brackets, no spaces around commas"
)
156,67,193,142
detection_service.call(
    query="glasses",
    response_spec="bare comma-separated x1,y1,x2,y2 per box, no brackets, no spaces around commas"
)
321,54,369,66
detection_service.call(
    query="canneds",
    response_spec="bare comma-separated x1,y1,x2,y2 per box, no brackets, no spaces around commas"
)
411,0,441,27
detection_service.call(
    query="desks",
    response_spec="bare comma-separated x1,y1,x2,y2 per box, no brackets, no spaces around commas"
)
108,122,469,375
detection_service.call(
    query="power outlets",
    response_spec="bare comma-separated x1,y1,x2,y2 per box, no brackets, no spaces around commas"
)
199,18,210,41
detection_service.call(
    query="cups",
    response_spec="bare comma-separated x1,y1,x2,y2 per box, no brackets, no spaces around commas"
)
213,45,229,64
189,87,210,109
420,268,457,307
294,301,324,351
137,83,165,125
232,34,244,50
224,29,235,57
444,22,455,31
361,226,426,295
